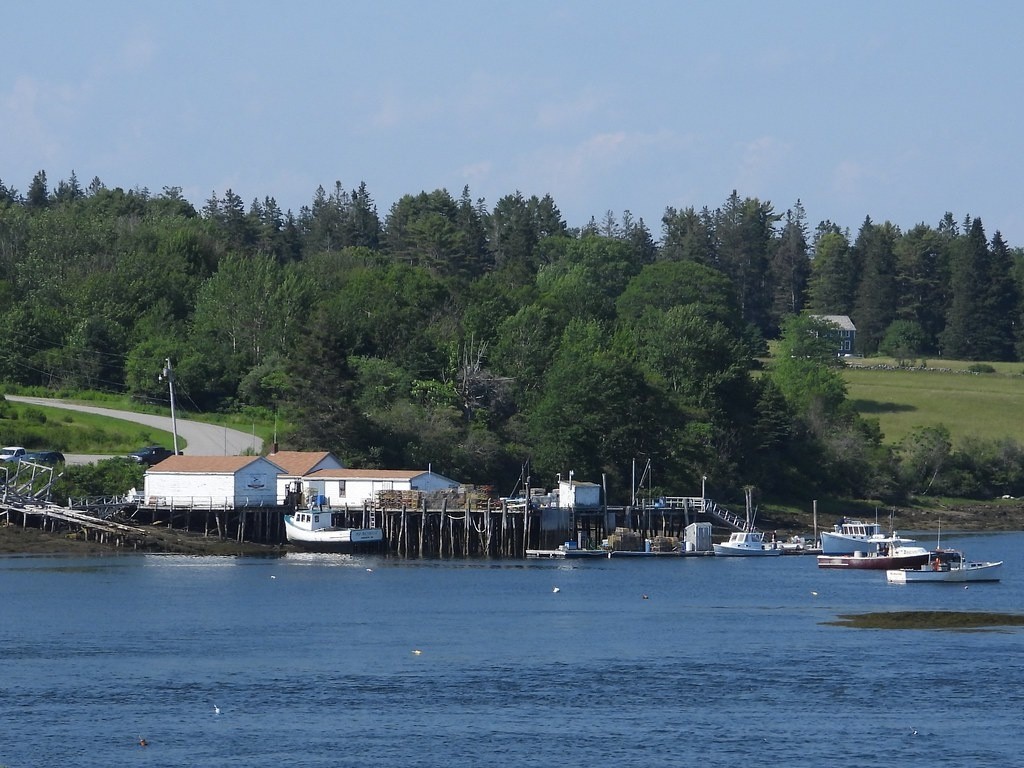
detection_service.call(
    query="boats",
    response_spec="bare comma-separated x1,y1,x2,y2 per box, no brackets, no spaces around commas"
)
780,499,918,554
284,504,384,551
816,537,965,570
886,516,1004,583
712,487,781,556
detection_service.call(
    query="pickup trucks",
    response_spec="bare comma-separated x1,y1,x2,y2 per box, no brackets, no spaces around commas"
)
129,446,184,465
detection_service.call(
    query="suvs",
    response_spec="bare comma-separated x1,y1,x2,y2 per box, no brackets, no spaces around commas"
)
0,446,28,465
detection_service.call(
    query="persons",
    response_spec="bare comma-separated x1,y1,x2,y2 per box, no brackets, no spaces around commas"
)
772,530,776,542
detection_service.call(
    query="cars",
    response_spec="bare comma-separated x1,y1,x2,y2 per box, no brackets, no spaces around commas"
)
29,450,65,466
